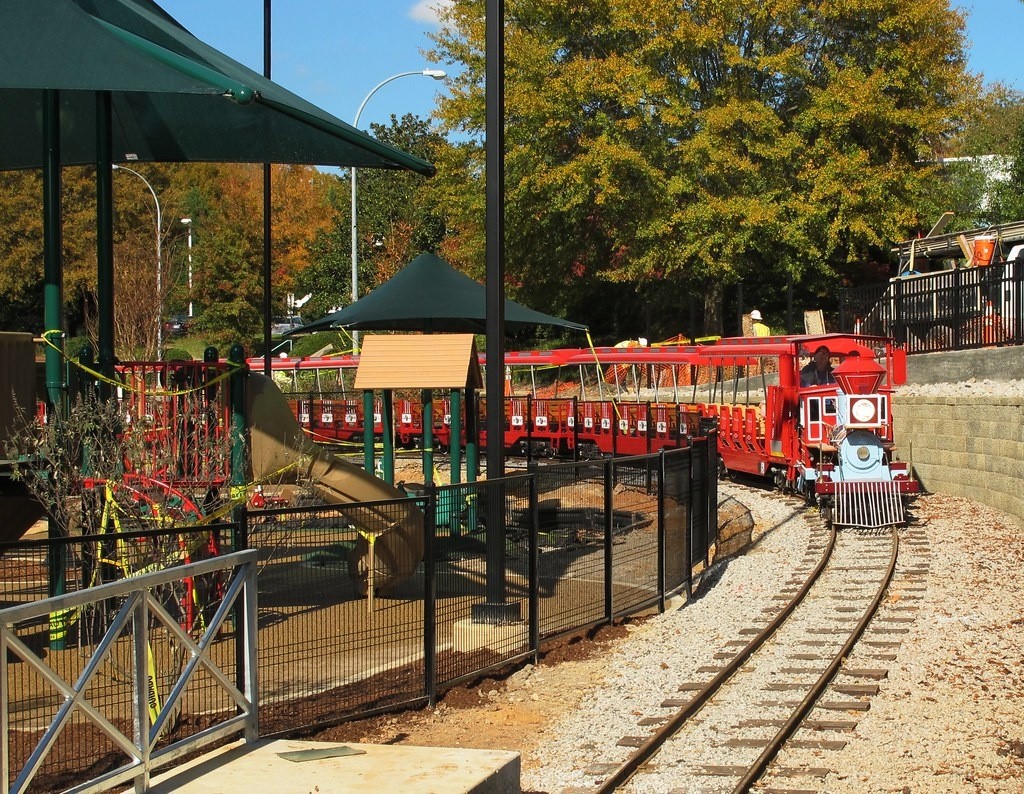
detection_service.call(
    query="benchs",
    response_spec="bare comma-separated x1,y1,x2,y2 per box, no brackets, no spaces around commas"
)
286,398,765,440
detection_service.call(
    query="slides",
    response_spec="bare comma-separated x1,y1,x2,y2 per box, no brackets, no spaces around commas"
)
249,433,425,594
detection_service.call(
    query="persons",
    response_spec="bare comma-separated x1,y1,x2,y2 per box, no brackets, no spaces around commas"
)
798,346,837,415
749,310,770,337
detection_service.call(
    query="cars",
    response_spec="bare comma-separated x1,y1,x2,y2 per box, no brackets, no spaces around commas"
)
161,315,190,335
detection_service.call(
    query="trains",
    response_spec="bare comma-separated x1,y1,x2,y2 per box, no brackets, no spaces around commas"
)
117,333,922,529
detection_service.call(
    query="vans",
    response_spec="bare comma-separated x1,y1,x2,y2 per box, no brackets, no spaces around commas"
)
1001,245,1024,339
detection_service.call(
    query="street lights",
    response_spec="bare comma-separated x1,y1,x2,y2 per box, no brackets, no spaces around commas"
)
352,70,447,355
181,218,194,320
111,164,161,388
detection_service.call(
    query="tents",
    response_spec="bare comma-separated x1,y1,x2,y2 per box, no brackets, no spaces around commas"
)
1,1,437,649
281,253,590,539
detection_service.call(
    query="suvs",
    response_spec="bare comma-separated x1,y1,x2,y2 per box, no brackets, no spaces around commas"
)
272,316,318,336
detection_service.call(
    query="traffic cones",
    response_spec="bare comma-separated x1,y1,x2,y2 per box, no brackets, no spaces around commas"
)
982,301,998,347
